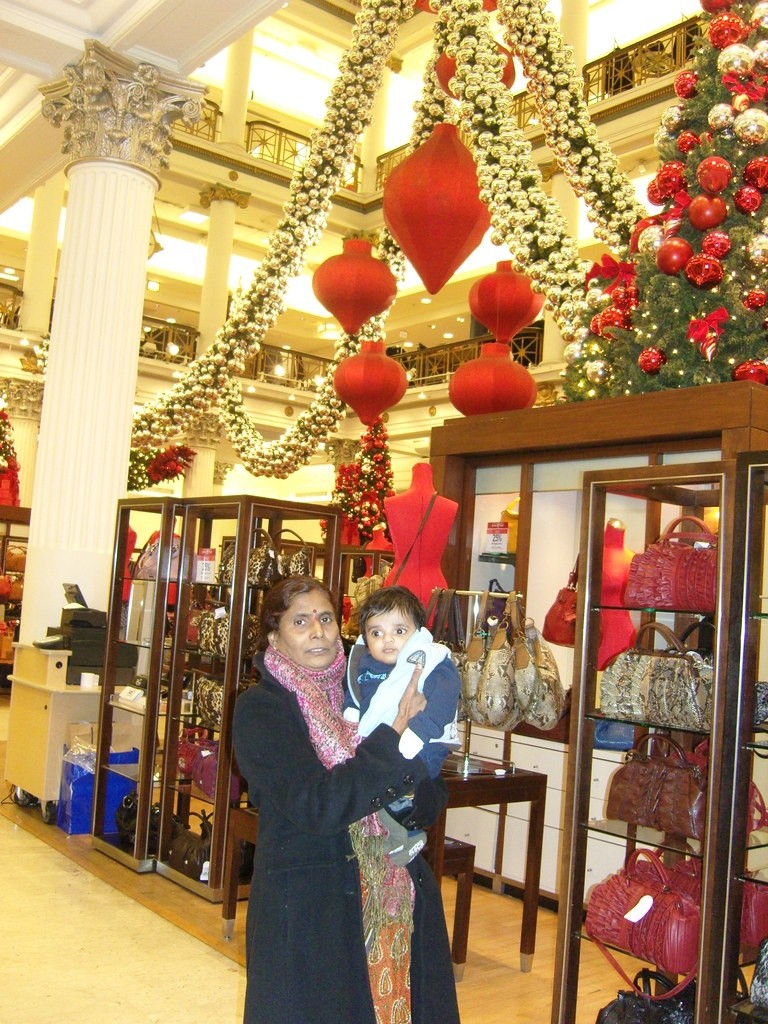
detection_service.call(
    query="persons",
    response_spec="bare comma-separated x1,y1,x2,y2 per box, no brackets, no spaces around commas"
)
596,518,640,667
384,462,459,630
343,586,461,872
233,577,460,1024
123,517,183,606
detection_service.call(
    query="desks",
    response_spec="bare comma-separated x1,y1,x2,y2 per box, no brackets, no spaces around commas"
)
429,753,549,953
221,807,477,984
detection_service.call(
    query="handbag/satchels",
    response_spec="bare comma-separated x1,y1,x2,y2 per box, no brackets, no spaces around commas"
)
0,546,29,688
542,554,580,648
583,515,768,1024
113,528,313,880
421,579,566,731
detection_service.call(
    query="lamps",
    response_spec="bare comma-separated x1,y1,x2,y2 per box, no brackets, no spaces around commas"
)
407,368,420,388
168,342,179,356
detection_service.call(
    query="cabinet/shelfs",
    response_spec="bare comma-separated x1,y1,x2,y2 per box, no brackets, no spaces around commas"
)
91,495,396,905
0,537,29,695
429,380,768,1024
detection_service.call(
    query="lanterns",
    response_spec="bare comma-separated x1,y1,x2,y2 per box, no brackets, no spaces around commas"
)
312,239,397,335
383,124,492,295
469,262,547,341
449,343,538,415
335,342,408,426
436,34,515,100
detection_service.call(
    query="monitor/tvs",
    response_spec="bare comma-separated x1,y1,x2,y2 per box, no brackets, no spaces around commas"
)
62,583,88,609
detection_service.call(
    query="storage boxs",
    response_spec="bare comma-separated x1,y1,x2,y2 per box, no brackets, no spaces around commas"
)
58,743,138,835
